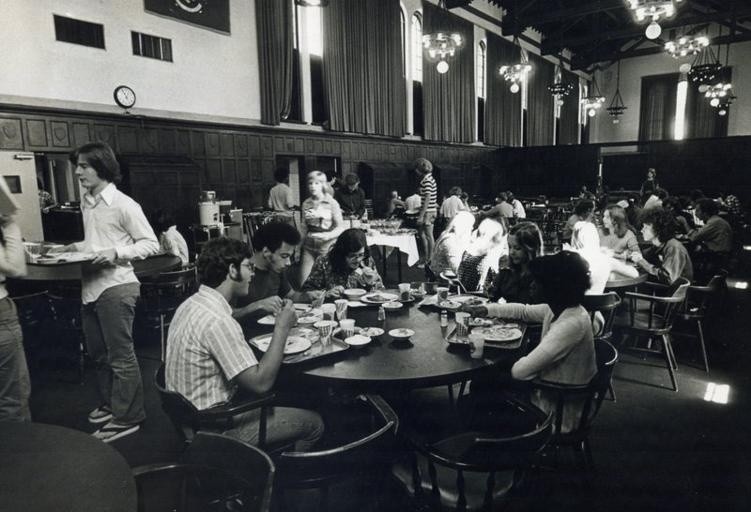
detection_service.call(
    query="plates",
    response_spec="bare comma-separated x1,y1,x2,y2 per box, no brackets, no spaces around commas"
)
440,301,462,310
297,307,323,321
471,325,521,342
257,335,312,354
361,293,398,303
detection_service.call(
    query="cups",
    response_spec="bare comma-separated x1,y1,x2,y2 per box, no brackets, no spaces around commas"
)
335,299,348,323
470,335,484,359
318,321,332,346
399,283,410,300
321,303,336,322
455,312,471,337
341,318,355,338
437,288,449,305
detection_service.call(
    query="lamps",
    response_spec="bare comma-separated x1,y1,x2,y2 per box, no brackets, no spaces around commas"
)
627,1,738,116
607,59,627,125
546,52,573,106
420,0,464,74
498,34,532,93
580,63,606,117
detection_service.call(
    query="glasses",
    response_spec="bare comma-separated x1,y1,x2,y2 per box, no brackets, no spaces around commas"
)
238,263,256,272
345,253,366,262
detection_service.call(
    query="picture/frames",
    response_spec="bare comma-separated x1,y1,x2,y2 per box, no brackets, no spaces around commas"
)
143,0,231,36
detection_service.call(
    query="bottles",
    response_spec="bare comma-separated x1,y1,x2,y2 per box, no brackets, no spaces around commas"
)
441,310,448,328
378,307,386,321
361,209,369,234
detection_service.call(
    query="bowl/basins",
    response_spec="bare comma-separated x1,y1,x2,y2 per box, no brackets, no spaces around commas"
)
358,327,386,339
344,335,371,348
343,288,366,301
382,302,402,311
387,327,414,343
411,288,426,300
314,320,338,328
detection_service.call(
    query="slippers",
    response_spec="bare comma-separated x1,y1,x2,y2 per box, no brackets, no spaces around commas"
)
91,423,140,443
88,404,112,423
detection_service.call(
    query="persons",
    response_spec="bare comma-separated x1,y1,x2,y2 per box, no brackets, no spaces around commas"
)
644,166,662,191
300,170,344,290
426,184,737,479
159,211,189,264
297,228,386,295
385,191,407,226
37,145,159,444
231,221,325,329
341,172,365,222
267,174,295,214
413,158,438,269
1,177,33,450
163,239,301,509
406,189,421,221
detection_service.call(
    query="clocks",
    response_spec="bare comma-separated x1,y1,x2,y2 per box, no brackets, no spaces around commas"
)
113,85,136,109
